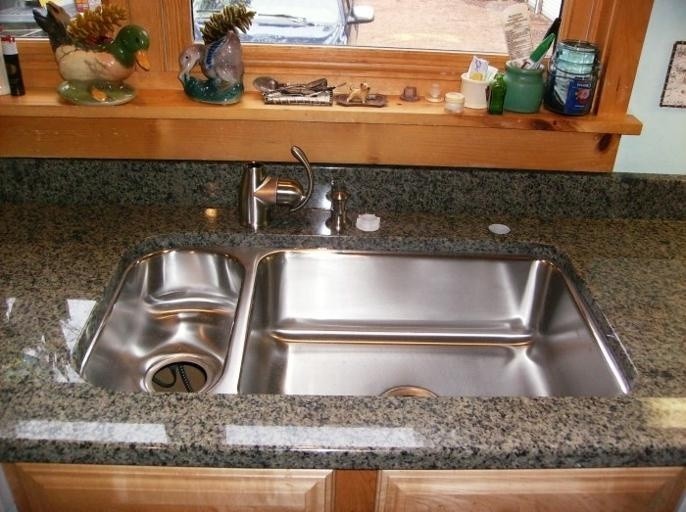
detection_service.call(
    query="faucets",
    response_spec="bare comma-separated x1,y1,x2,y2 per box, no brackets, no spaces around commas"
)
238,144,316,233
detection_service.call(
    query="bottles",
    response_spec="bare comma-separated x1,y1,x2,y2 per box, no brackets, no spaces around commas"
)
487,73,507,115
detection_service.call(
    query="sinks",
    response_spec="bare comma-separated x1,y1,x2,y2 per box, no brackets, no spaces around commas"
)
64,228,250,403
227,239,647,408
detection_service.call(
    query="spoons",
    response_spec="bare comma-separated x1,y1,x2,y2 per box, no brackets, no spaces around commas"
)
252,76,309,93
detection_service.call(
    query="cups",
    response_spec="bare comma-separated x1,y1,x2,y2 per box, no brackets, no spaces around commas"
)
6,35,26,96
444,92,466,115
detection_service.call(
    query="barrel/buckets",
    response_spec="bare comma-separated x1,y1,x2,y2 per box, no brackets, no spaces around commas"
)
543,40,600,115
